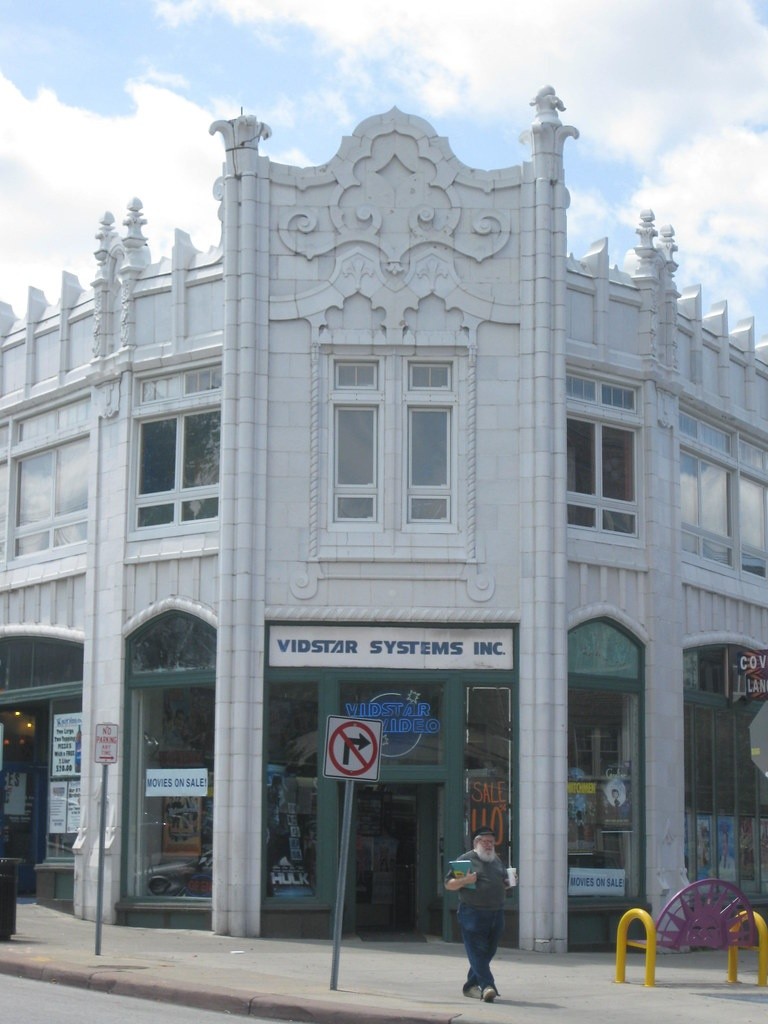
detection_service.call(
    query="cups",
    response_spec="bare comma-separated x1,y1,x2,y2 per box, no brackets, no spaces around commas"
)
507,868,517,886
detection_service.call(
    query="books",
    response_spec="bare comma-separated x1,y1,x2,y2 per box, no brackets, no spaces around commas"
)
448,860,476,889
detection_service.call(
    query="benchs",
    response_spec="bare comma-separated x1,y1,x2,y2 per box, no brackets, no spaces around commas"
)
615,878,768,986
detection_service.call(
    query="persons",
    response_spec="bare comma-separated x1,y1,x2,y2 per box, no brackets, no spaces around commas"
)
165,796,197,840
696,825,737,882
443,826,519,1003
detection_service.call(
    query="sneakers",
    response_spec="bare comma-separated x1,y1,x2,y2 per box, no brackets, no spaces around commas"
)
482,987,497,1003
463,985,483,999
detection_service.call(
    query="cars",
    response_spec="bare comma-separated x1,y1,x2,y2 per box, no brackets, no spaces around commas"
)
143,849,213,896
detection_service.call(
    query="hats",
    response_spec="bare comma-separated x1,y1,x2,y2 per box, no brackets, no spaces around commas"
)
472,827,496,840
